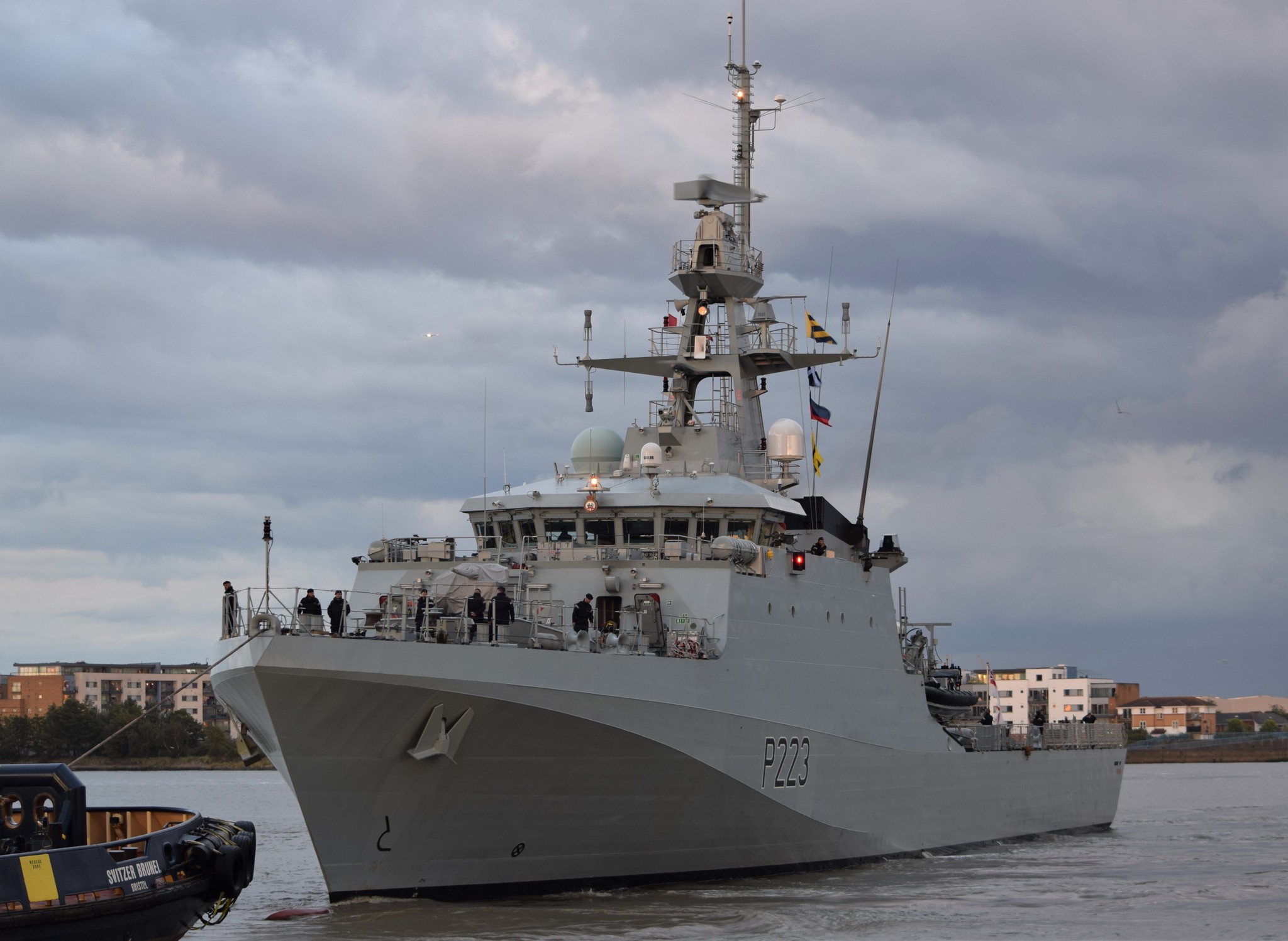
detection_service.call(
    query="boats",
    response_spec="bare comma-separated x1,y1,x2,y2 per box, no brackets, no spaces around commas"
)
210,0,1126,902
0,763,256,941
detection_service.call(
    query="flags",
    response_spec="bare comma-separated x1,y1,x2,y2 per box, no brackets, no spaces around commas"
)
812,431,823,477
806,365,821,388
806,311,837,345
809,393,832,426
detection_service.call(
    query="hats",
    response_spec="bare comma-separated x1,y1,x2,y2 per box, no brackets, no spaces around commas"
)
586,593,593,600
421,589,427,593
307,588,314,592
223,581,230,585
335,591,341,595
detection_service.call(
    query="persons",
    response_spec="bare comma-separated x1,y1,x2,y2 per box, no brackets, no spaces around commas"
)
572,594,594,632
297,589,322,615
415,590,435,642
223,580,238,638
487,587,515,643
811,537,826,556
328,591,351,637
467,590,486,644
